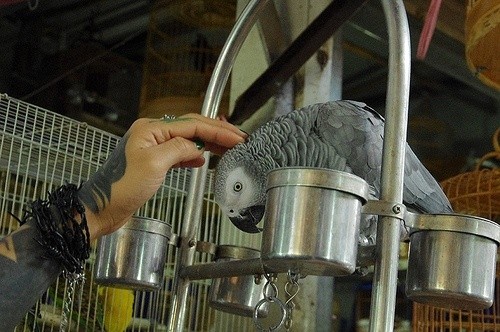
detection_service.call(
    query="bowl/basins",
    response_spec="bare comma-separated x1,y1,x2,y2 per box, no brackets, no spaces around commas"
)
207,244,276,318
259,166,370,276
94,216,181,292
405,214,500,312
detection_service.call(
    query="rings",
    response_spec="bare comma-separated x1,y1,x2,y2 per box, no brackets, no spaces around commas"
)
160,113,176,120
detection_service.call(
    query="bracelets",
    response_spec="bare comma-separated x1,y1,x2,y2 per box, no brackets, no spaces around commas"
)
13,177,94,276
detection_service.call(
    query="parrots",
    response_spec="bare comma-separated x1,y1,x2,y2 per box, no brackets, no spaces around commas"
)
213,99,455,276
